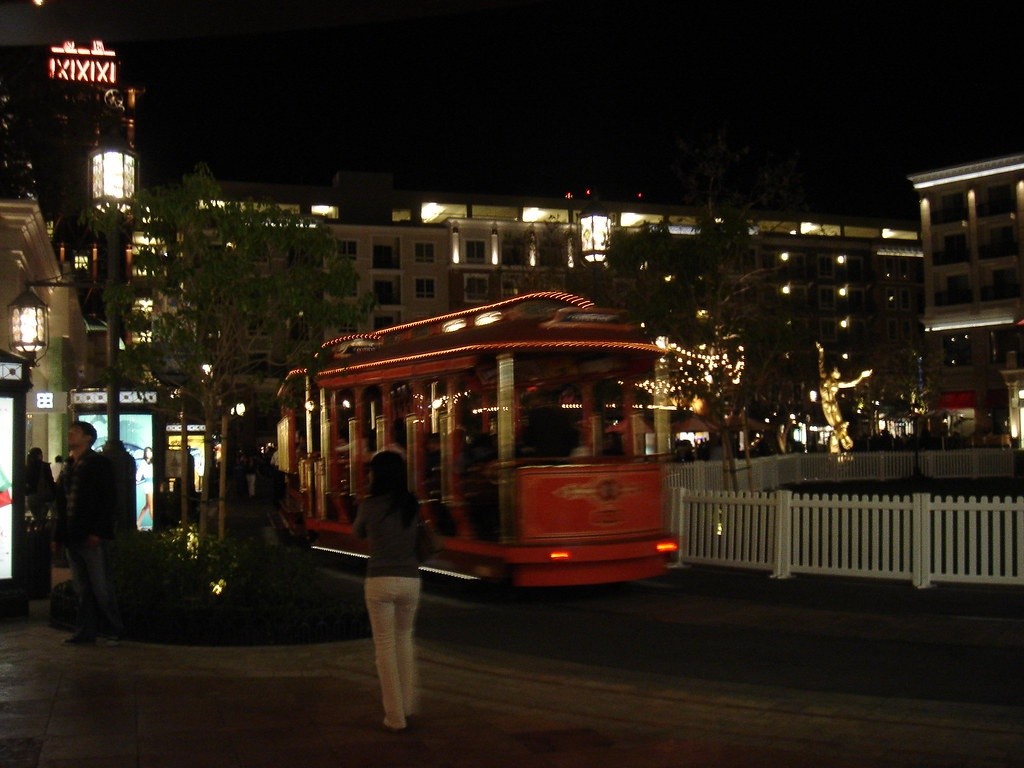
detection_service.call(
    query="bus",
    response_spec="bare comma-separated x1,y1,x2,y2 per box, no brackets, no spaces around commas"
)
276,288,681,589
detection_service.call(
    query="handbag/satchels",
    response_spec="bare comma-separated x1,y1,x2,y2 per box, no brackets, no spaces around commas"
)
38,464,56,501
413,505,443,564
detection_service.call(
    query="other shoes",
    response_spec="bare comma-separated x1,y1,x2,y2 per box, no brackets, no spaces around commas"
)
66,636,96,644
382,720,409,734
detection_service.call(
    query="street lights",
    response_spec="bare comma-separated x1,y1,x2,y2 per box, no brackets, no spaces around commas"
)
6,81,146,528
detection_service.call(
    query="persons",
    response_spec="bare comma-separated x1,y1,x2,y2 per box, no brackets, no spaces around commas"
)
351,452,425,732
816,340,873,454
296,390,625,506
23,445,63,526
51,421,129,644
